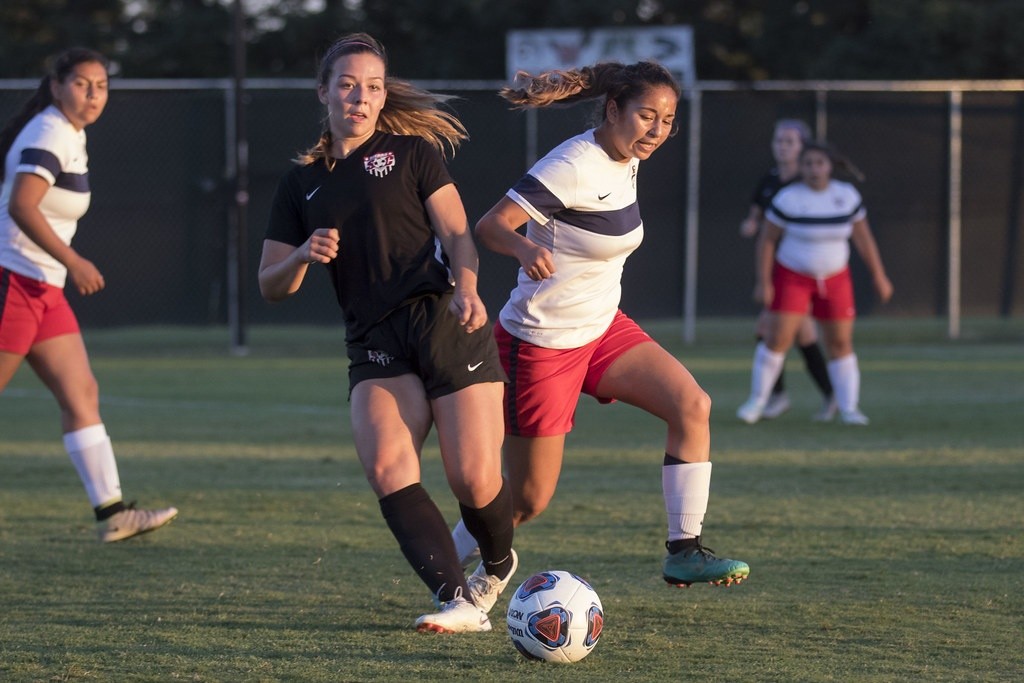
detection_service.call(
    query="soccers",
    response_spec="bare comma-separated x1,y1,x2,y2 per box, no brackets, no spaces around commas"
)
505,568,605,664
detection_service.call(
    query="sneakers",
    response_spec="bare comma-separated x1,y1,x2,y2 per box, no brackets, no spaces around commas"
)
662,536,750,587
466,548,518,614
414,586,492,633
97,500,178,544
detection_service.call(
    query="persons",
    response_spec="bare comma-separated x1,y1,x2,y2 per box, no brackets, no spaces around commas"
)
258,31,520,636
443,57,750,588
735,120,896,427
0,47,182,544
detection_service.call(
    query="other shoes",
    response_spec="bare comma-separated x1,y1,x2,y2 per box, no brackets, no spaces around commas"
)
841,410,870,426
816,397,836,422
736,402,764,424
763,393,790,419
